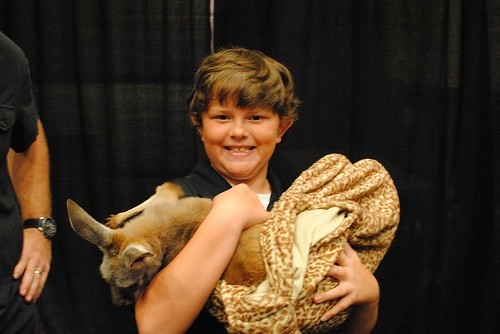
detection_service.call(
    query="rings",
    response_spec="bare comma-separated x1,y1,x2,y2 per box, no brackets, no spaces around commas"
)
35,270,43,274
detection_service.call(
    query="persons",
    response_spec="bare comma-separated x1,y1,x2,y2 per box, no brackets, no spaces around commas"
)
0,30,57,334
134,48,381,334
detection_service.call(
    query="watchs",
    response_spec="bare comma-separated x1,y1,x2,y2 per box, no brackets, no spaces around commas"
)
23,217,57,238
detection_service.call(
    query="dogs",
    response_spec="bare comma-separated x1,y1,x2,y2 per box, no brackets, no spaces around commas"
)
66,181,271,309
